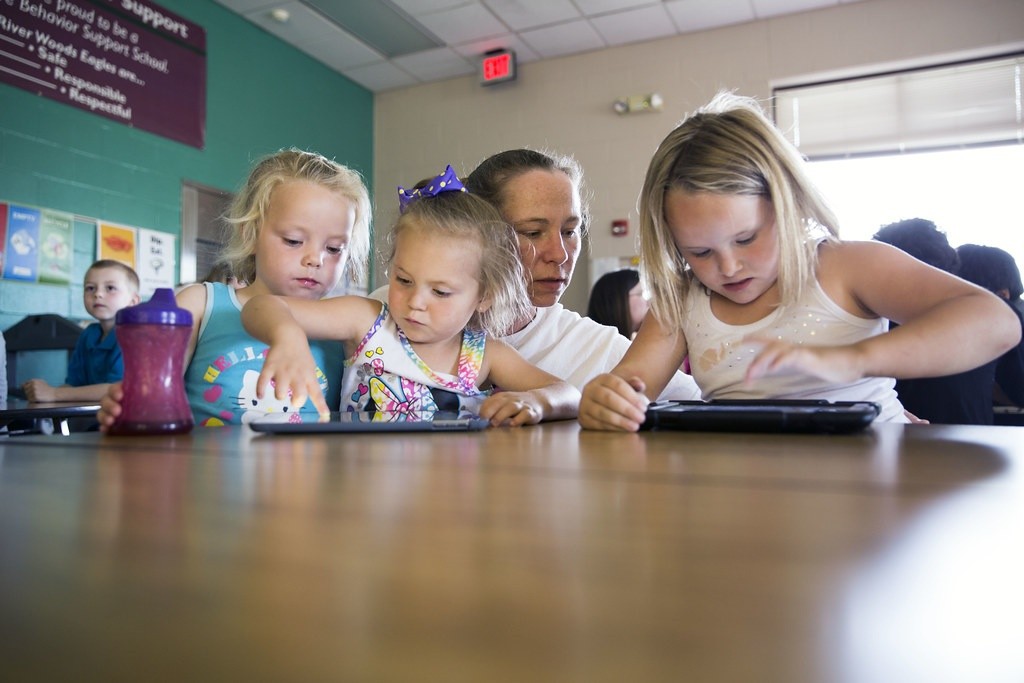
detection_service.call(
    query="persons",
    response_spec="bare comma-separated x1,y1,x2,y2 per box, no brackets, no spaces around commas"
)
954,242,1024,411
868,218,996,424
577,97,1021,427
363,149,704,401
93,147,374,434
587,268,651,341
22,261,143,403
239,165,584,431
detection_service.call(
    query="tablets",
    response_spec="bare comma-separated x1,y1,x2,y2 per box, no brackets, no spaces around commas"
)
249,412,490,434
639,398,881,433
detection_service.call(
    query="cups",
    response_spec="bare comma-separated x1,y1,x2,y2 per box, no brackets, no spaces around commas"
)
108,286,194,436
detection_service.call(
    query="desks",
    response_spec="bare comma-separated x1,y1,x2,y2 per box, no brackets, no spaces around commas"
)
0,422,1023,682
0,401,104,419
